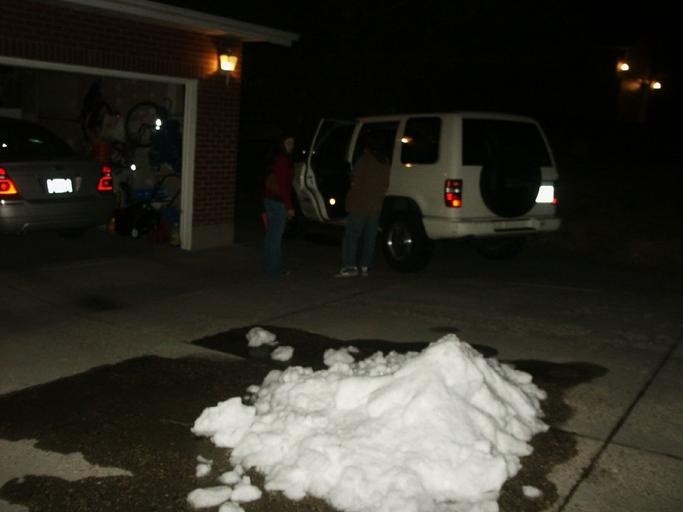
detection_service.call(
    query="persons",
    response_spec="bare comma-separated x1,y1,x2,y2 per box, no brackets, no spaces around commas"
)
334,138,393,278
256,132,299,278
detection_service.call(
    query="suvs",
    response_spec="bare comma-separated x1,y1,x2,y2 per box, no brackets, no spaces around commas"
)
289,112,561,269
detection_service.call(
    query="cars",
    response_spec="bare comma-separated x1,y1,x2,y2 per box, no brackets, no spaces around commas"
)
1,117,116,239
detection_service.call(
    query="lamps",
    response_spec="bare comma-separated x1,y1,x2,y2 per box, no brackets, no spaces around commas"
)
218,53,237,87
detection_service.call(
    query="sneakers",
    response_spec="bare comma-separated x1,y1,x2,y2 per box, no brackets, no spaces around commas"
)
334,266,368,277
